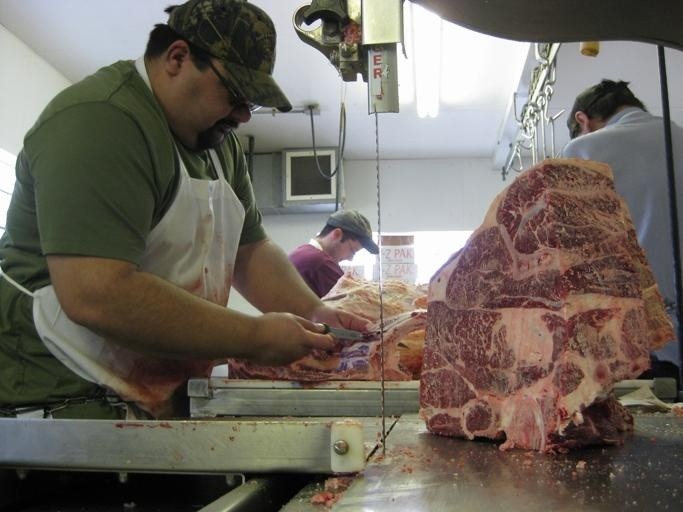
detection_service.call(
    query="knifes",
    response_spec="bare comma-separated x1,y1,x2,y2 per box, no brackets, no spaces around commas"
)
323,322,363,341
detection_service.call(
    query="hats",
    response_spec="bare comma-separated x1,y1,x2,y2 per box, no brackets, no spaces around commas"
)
164,0,295,113
565,78,635,140
325,209,379,255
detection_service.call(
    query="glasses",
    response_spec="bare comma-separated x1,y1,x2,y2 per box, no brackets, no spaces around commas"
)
195,48,264,113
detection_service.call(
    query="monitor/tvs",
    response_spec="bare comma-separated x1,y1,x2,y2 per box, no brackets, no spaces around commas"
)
286,151,336,199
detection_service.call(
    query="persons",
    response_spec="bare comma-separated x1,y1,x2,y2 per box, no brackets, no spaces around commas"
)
287,206,380,296
0,0,380,511
560,79,682,389
